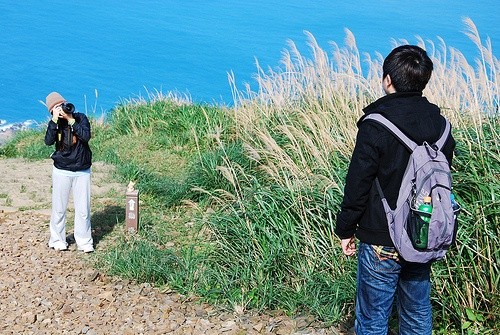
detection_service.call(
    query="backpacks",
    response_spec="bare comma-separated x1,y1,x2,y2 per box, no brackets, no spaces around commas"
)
363,113,460,264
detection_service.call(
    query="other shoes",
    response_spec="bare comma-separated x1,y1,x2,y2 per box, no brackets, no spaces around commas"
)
84,248,94,253
54,245,67,250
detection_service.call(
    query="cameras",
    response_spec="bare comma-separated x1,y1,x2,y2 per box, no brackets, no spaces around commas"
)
58,103,75,116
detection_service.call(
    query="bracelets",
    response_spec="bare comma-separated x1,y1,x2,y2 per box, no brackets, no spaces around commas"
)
51,118,58,123
68,119,75,125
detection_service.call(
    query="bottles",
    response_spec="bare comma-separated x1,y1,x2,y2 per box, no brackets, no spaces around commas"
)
412,196,432,248
450,194,459,210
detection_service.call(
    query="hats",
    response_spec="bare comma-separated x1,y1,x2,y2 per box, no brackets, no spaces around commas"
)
46,92,67,115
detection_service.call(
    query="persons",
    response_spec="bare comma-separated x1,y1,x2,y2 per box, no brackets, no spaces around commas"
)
44,92,94,253
336,45,456,335
